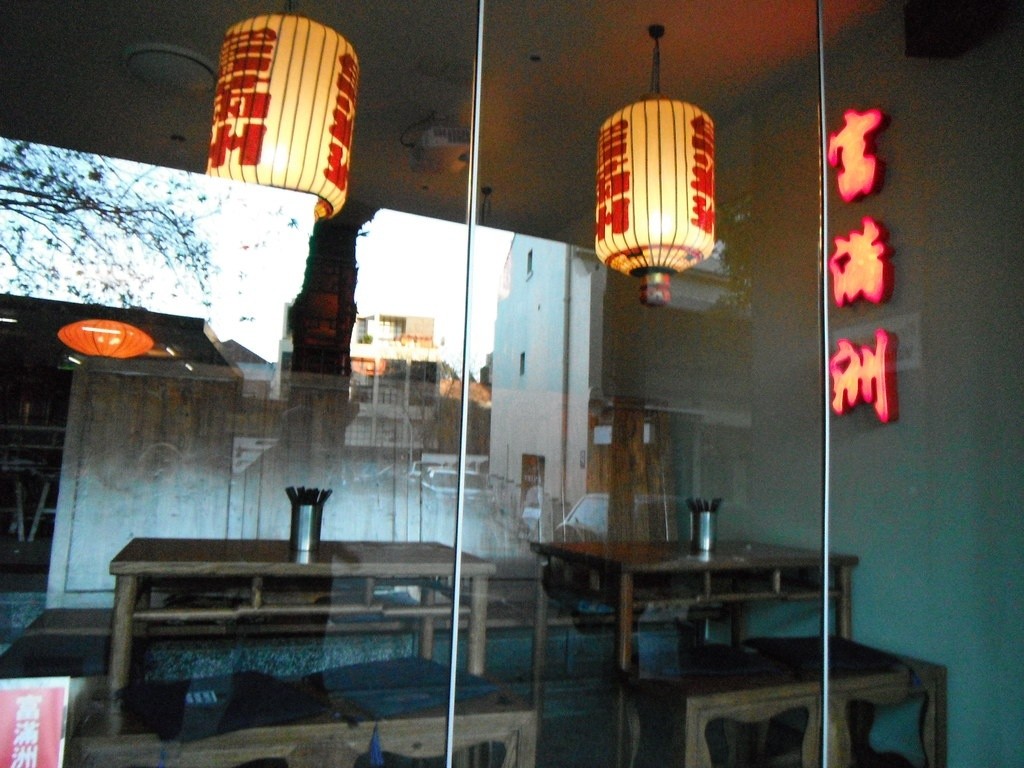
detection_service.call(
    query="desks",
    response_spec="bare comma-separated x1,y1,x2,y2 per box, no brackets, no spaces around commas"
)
0,463,62,543
530,537,860,673
102,532,498,708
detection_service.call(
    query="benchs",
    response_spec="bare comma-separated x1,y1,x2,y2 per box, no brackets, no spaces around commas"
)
0,606,114,768
73,654,539,768
614,635,949,768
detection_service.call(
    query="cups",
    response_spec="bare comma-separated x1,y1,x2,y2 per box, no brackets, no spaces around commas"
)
290,505,321,553
691,510,716,551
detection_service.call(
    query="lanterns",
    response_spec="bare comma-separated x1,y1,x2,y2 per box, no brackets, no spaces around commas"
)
594,100,717,308
204,15,360,224
57,320,155,359
